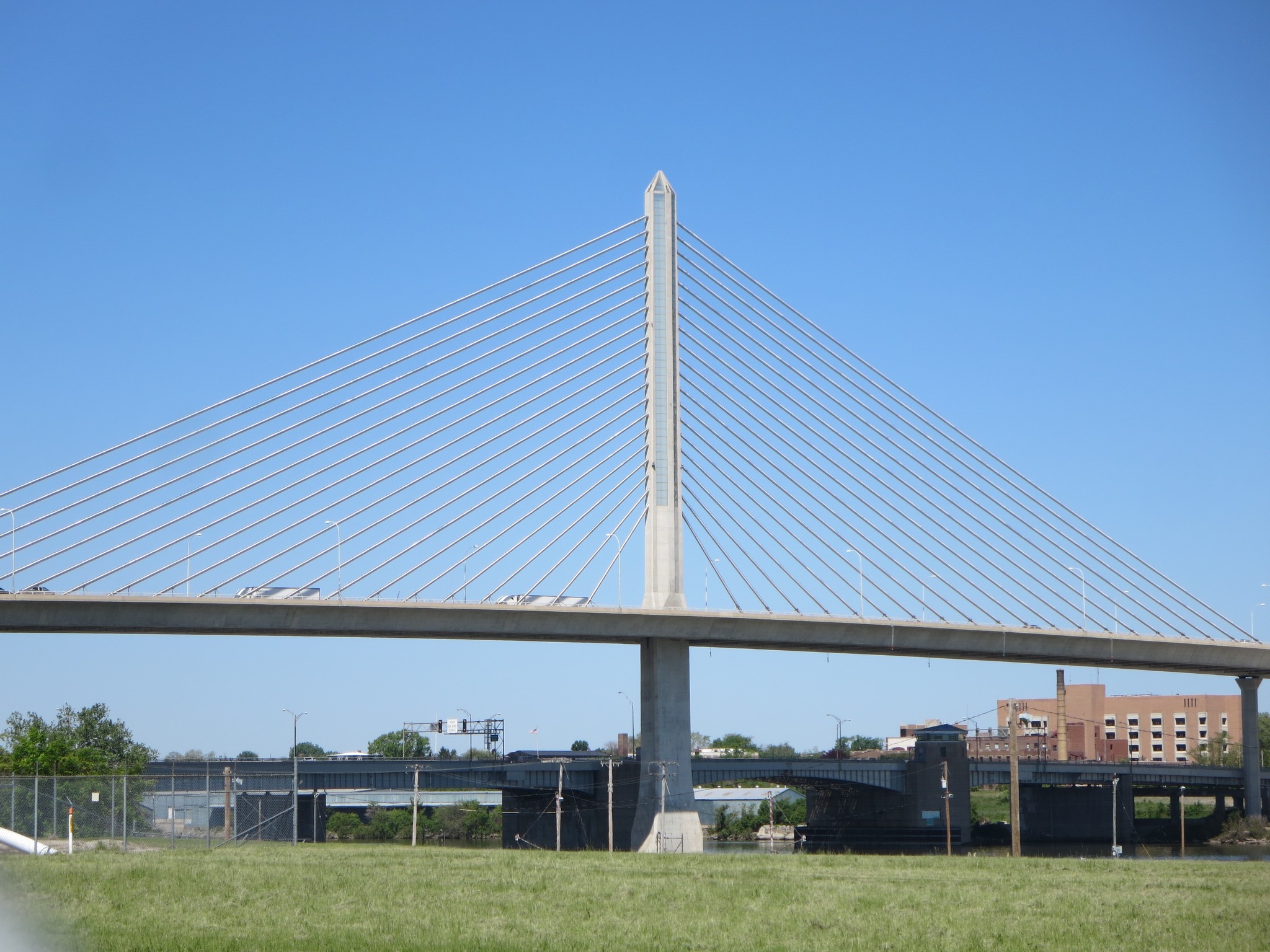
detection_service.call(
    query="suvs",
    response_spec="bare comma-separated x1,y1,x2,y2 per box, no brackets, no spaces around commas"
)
1240,639,1251,643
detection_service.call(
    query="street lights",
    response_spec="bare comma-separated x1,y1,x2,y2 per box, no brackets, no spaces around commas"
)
921,574,938,622
845,549,865,617
1114,590,1129,634
283,709,308,760
619,691,635,760
1,507,15,594
704,558,720,612
463,544,478,604
826,713,851,760
325,519,342,599
605,534,621,607
457,708,501,760
1069,566,1086,630
1250,602,1266,642
186,531,203,597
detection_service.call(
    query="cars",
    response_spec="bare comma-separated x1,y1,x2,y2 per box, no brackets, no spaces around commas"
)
0,587,10,594
28,586,57,595
302,756,316,761
517,756,541,763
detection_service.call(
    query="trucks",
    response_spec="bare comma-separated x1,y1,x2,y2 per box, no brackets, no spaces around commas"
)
495,595,589,608
234,587,321,601
